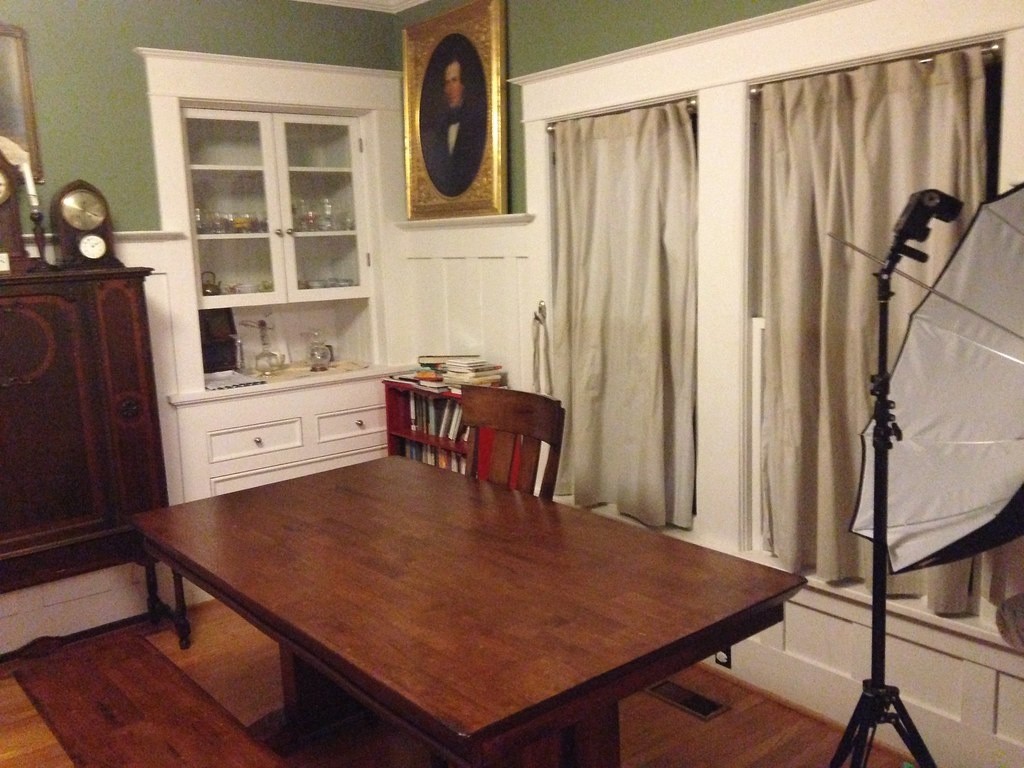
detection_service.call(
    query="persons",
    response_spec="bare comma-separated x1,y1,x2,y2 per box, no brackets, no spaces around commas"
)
429,57,485,196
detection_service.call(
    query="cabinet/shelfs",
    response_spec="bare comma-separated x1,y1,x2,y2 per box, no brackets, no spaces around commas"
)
180,97,373,310
167,358,418,504
382,377,516,486
1,265,169,595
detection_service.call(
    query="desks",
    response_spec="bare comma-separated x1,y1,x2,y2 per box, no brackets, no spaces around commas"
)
130,458,808,768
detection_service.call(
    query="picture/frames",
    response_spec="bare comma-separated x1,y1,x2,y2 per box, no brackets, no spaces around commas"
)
401,0,510,220
0,21,45,184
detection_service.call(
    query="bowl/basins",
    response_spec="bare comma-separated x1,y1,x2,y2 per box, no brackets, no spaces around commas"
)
235,285,259,293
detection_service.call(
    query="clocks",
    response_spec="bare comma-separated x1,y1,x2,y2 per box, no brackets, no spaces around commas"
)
49,178,126,271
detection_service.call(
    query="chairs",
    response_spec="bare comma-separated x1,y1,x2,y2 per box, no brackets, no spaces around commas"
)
461,383,566,500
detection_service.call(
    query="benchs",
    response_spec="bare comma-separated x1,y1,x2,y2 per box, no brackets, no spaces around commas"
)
12,627,289,768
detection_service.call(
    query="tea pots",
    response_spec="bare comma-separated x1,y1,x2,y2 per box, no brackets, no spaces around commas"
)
201,271,222,296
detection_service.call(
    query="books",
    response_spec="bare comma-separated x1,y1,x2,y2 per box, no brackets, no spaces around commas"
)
405,390,471,474
418,355,503,394
